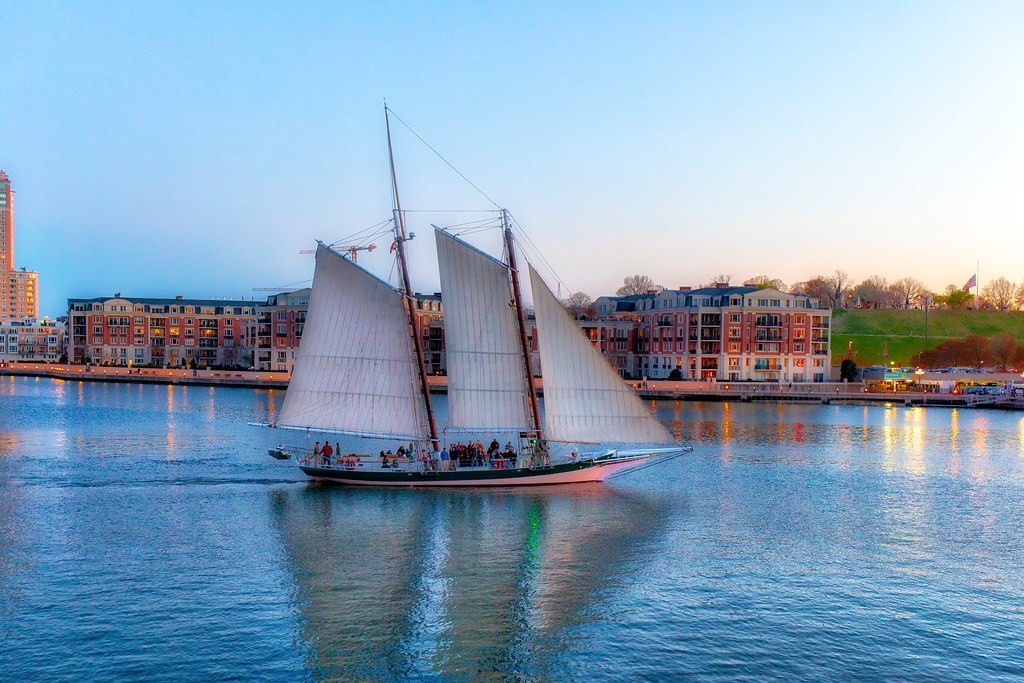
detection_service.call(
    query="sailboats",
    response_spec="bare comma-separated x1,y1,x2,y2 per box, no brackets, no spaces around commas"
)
245,84,695,492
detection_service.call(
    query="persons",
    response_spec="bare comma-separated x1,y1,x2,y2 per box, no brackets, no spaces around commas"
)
313,438,519,468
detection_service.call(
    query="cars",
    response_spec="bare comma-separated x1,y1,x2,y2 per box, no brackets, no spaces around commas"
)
863,366,1024,402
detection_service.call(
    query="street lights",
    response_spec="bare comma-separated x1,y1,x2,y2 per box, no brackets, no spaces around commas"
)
918,351,923,366
